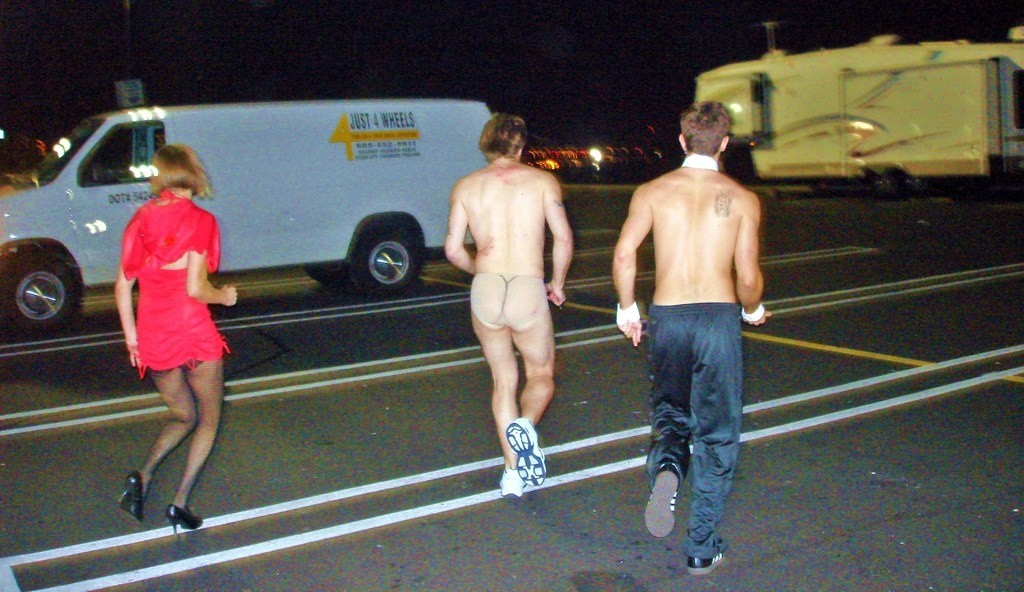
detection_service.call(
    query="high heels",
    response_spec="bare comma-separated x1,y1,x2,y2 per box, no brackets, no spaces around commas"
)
166,504,202,539
117,470,143,523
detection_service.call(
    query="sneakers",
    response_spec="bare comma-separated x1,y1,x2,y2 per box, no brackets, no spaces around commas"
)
688,551,726,575
500,468,526,498
644,462,681,537
505,416,545,489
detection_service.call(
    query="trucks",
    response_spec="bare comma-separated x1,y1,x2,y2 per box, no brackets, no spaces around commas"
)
691,19,1023,202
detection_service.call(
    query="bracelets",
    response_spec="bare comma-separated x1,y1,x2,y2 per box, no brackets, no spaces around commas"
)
615,301,641,324
741,303,765,323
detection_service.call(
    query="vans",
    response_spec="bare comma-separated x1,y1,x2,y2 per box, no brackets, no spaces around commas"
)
1,97,501,338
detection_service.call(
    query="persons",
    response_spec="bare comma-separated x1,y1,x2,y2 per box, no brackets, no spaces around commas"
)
445,111,576,501
112,143,238,542
612,99,772,576
